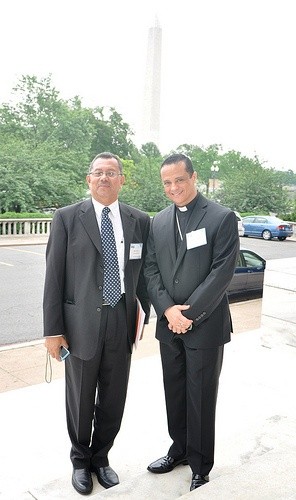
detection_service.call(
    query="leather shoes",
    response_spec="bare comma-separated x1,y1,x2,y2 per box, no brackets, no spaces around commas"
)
96,465,119,488
72,469,93,494
190,473,208,491
148,455,189,473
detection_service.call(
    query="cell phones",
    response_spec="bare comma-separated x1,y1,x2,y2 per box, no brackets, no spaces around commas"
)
60,345,69,360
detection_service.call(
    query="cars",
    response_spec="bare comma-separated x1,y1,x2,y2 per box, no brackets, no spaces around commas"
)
240,215,294,241
232,210,245,238
227,249,267,304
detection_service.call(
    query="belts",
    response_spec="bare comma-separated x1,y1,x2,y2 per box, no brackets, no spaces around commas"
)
120,294,125,302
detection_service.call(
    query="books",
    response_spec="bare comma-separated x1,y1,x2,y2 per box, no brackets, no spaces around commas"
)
135,296,146,349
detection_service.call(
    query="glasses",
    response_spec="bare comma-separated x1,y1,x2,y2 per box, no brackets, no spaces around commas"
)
89,170,121,178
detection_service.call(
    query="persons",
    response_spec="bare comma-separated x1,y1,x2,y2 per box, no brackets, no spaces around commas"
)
42,154,151,495
145,154,240,491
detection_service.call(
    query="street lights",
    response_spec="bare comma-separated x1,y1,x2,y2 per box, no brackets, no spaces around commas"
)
210,164,219,194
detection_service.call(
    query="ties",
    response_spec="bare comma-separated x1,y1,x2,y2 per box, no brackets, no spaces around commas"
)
101,207,121,309
